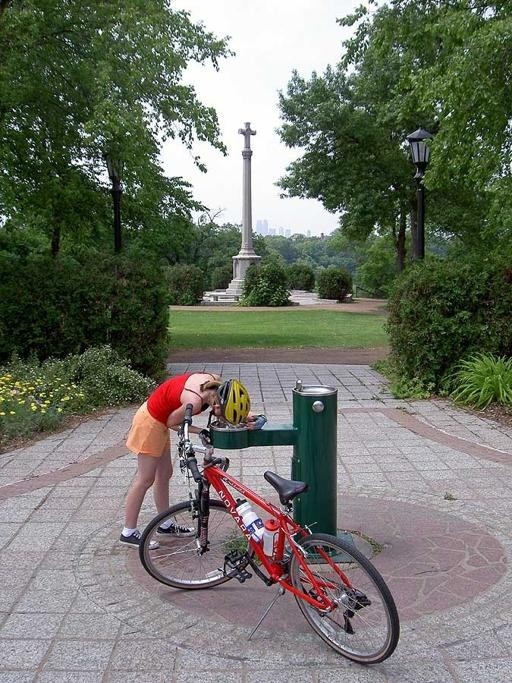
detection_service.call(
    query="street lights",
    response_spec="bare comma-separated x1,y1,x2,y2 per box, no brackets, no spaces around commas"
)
102,139,128,254
406,127,438,258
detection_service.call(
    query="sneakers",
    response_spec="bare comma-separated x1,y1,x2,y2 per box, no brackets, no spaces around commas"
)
119,530,160,550
156,523,198,537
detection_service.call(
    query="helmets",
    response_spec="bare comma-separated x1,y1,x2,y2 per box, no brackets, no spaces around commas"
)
216,379,250,426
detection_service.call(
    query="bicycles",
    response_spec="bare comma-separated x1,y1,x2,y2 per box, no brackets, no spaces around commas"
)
138,402,401,664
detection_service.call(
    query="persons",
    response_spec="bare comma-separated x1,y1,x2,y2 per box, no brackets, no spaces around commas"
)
119,371,265,549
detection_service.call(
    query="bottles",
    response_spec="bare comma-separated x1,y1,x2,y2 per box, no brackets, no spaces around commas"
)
261,518,281,555
234,495,266,541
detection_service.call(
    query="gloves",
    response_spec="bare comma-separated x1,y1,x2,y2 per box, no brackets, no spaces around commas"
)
199,429,213,447
